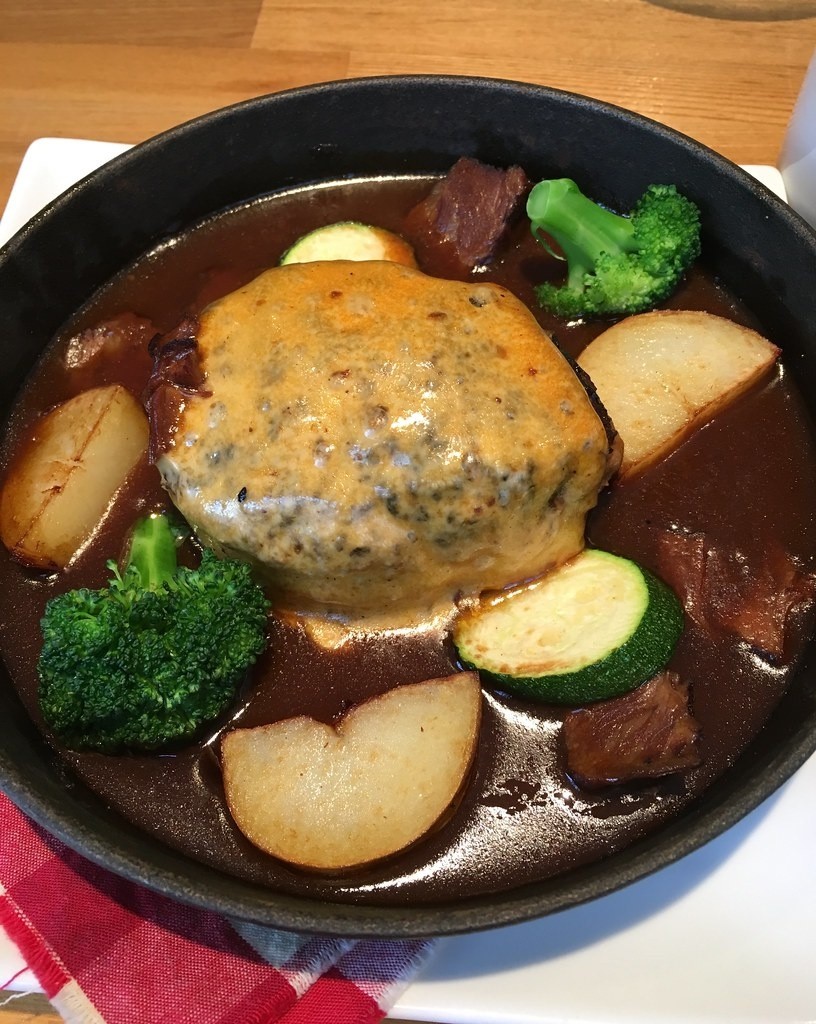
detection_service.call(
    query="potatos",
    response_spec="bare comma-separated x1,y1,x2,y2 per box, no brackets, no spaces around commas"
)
0,382,154,575
574,311,782,485
218,669,484,878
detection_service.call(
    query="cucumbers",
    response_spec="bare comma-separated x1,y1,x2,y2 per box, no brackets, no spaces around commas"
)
449,549,683,707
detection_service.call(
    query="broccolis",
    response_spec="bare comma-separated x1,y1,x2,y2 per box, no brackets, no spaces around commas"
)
35,515,272,756
526,177,700,321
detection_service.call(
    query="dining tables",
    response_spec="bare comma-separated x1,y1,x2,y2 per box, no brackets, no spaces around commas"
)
2,0,816,222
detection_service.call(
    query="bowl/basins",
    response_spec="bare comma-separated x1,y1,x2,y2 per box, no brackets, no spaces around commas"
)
3,71,814,947
